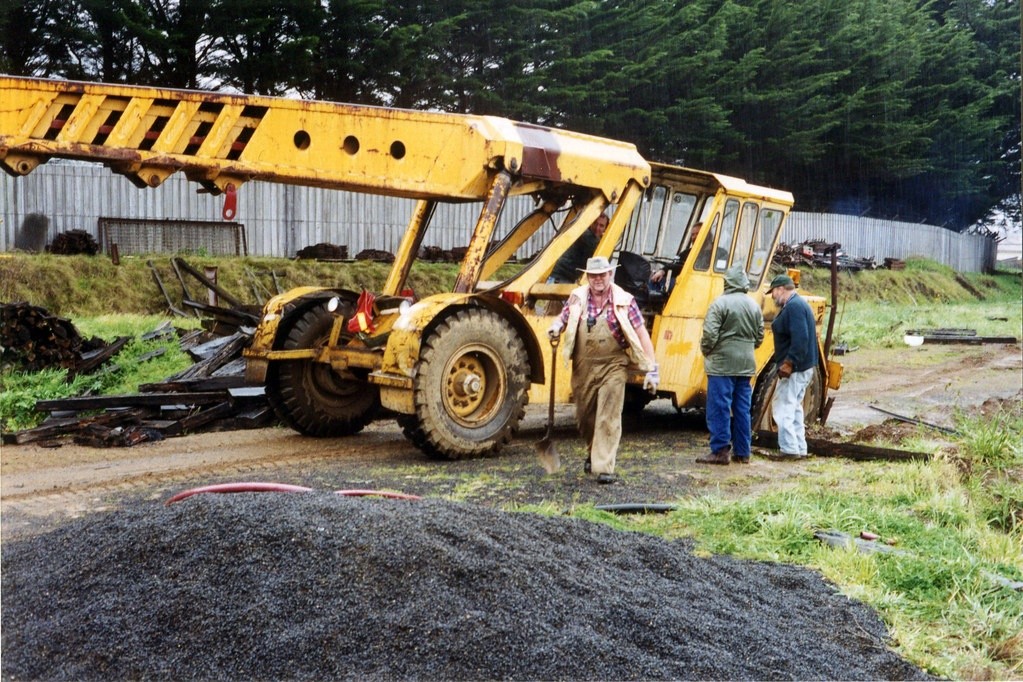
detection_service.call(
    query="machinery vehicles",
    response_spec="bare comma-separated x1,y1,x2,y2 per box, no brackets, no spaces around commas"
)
0,74,843,462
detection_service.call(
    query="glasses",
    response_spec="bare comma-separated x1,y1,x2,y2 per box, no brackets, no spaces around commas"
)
587,273,609,278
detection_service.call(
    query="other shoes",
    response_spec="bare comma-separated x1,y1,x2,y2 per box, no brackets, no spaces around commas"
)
583,456,591,474
695,446,730,465
596,473,614,484
732,454,749,464
768,454,808,461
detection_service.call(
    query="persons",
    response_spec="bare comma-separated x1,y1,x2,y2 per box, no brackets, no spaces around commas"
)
765,275,818,461
549,257,660,484
547,212,728,311
695,263,765,465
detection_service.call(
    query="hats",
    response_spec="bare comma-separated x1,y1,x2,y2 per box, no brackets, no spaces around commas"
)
576,257,621,274
765,274,794,295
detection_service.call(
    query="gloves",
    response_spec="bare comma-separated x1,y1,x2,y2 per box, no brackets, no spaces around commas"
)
546,323,563,339
642,364,658,394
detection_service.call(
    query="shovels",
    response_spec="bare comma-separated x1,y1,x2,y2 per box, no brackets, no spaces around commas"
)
534,330,561,474
